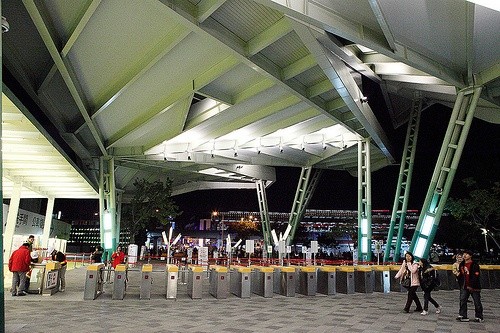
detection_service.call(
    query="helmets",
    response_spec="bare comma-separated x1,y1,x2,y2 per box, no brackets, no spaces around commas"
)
48,247,54,254
30,251,38,259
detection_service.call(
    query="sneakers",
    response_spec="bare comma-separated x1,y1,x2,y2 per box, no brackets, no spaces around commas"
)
420,310,429,316
456,315,469,321
414,308,422,311
60,289,65,292
12,293,27,296
473,317,484,322
436,306,443,314
400,310,409,313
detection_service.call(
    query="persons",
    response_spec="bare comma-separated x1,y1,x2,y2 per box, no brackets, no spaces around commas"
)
25,235,34,252
111,245,124,269
453,251,484,322
50,248,67,292
395,252,422,313
8,242,32,296
146,246,384,260
91,245,104,282
418,258,442,316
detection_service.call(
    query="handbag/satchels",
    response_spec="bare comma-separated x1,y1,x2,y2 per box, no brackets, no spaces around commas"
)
403,279,411,287
